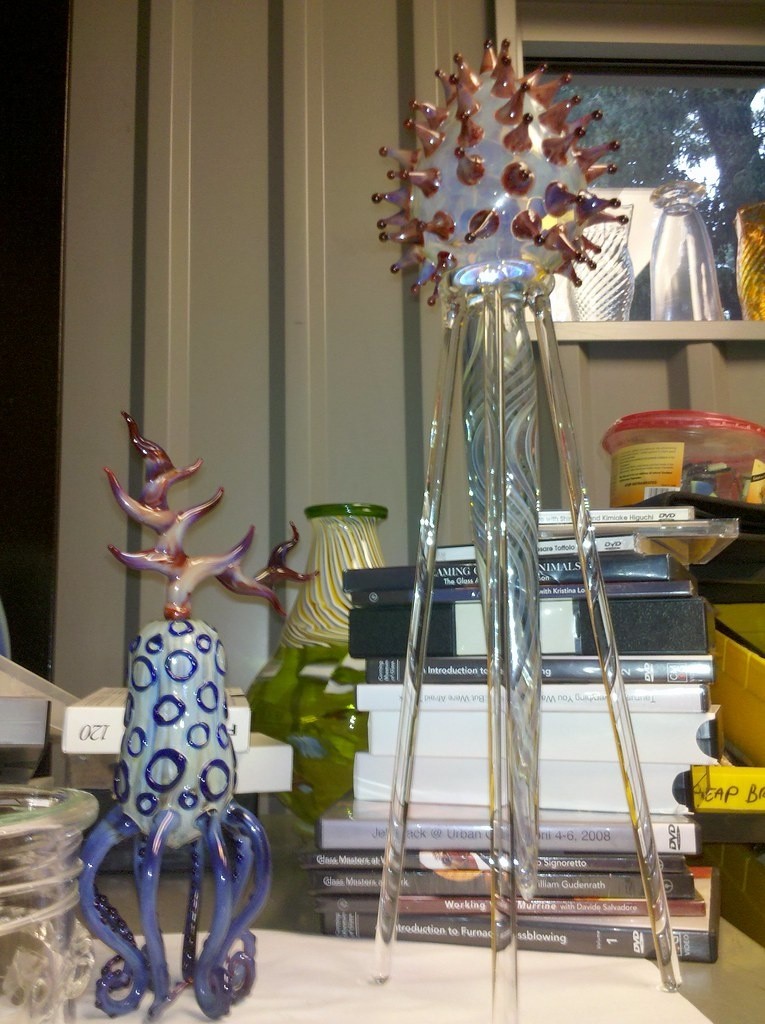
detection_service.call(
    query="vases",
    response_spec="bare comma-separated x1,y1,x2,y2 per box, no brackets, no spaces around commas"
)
570,206,632,322
250,504,387,832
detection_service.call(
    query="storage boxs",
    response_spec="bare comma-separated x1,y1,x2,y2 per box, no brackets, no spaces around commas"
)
712,604,764,767
61,685,295,875
691,840,764,948
0,654,64,781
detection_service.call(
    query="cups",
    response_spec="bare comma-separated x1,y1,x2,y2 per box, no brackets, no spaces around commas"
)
568,201,636,322
736,200,765,321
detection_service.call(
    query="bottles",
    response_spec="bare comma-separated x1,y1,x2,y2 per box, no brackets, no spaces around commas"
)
648,181,724,322
